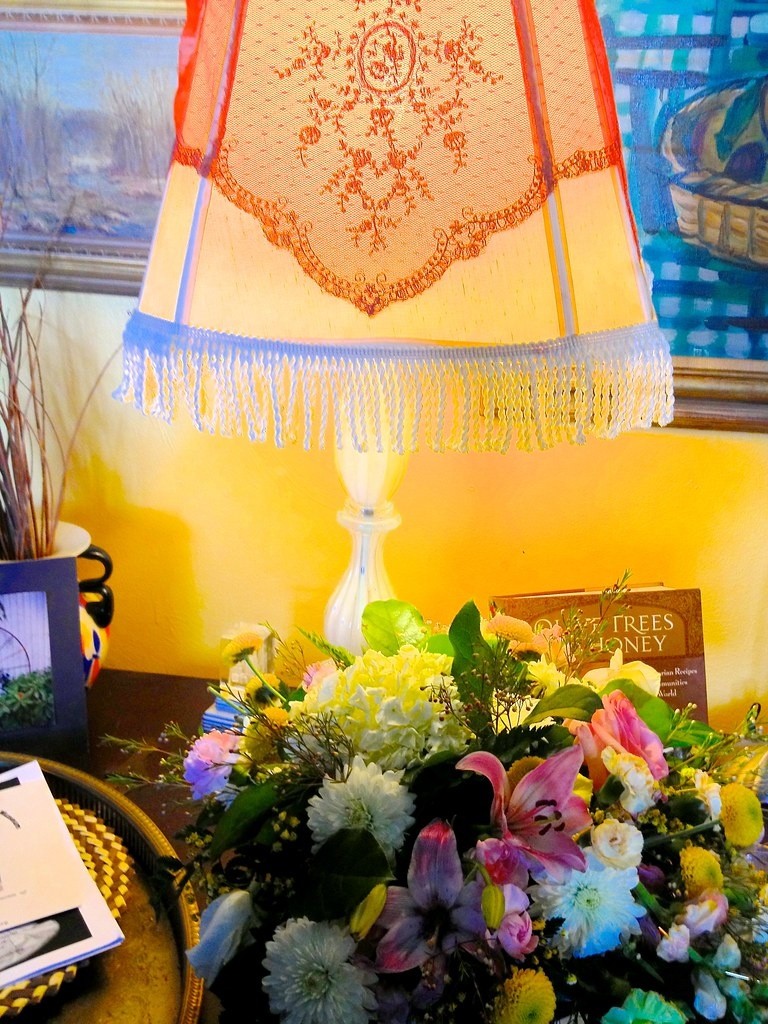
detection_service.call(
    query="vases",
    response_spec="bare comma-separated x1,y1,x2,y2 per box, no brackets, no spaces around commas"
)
0,521,115,689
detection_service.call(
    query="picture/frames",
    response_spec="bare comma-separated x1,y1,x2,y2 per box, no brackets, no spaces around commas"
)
0,556,89,739
478,0,768,431
0,7,186,297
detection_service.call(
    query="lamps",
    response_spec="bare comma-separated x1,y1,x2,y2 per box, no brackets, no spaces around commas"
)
110,0,675,665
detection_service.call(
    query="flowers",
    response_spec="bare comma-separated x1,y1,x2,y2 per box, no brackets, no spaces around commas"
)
96,571,768,1024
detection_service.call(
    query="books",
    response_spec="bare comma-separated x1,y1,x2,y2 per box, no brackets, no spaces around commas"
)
488,582,709,726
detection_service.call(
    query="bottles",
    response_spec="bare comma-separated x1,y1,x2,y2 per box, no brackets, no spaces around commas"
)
0,521,114,698
